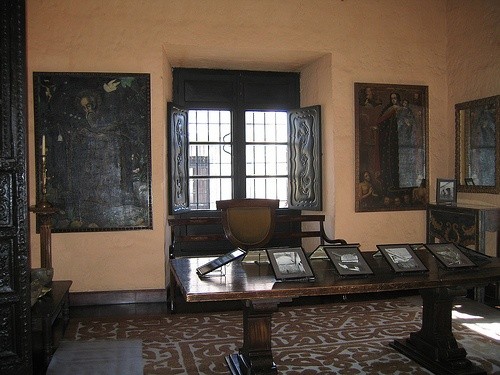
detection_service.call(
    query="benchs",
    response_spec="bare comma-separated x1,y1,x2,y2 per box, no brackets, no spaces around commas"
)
168,199,345,258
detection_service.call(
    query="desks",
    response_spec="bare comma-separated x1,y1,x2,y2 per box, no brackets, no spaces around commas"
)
169,249,500,375
29,279,73,364
427,203,500,257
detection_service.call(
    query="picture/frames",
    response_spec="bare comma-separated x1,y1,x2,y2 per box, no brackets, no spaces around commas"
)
456,95,500,192
436,178,458,205
197,243,491,281
353,82,429,213
32,70,153,233
